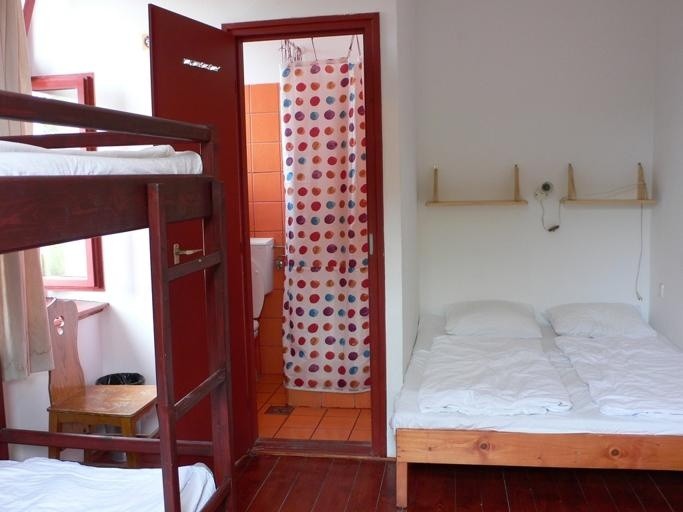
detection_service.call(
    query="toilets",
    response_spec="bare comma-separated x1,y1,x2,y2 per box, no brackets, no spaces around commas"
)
250,236,276,340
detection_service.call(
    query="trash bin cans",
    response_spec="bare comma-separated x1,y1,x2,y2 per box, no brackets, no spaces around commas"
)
95,372,145,462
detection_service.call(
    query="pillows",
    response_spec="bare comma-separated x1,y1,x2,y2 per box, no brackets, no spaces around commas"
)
444,301,656,338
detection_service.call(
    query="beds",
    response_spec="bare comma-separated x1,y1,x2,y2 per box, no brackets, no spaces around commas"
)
397,316,682,509
2,91,241,512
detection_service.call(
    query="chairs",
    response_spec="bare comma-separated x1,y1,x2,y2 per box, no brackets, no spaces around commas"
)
47,298,159,468
